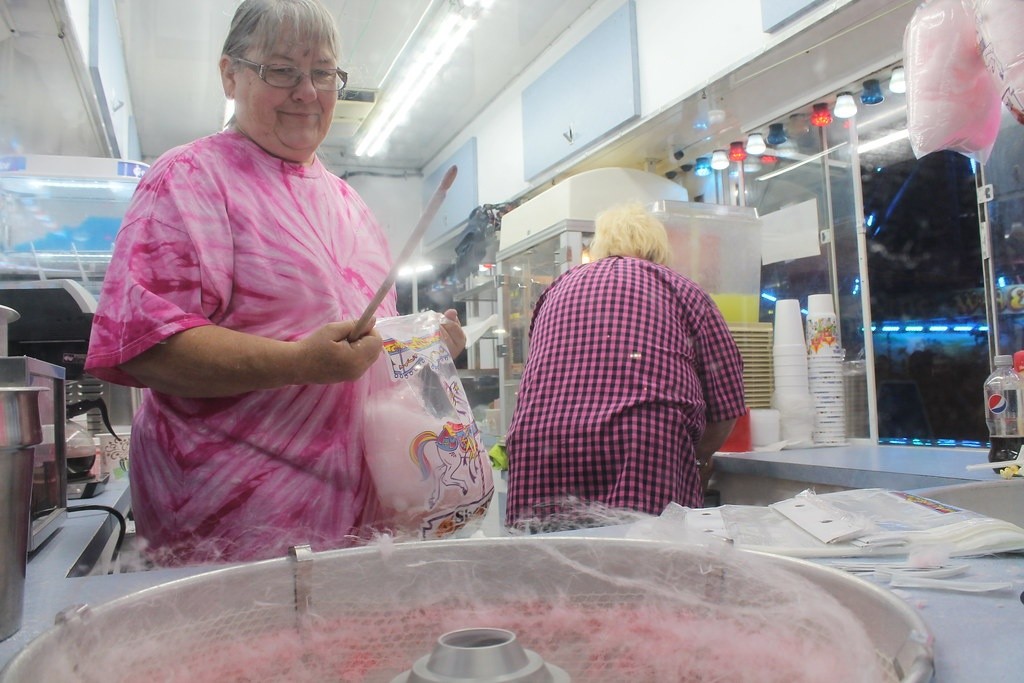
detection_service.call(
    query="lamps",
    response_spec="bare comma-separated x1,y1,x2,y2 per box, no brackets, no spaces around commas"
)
350,0,494,160
695,66,907,178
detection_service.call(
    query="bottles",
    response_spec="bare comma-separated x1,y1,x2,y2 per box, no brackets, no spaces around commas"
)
983,350,1024,475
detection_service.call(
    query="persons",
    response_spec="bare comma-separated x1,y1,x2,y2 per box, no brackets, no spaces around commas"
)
83,0,467,570
503,205,747,534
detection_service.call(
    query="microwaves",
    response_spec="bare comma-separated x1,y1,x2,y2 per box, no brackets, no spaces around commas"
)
4,354,74,559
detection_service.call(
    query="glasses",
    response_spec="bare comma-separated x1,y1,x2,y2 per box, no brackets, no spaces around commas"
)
232,57,350,91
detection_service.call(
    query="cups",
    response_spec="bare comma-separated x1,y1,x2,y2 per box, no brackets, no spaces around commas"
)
772,293,846,449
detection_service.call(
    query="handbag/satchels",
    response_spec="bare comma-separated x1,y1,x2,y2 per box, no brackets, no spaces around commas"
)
351,308,495,530
